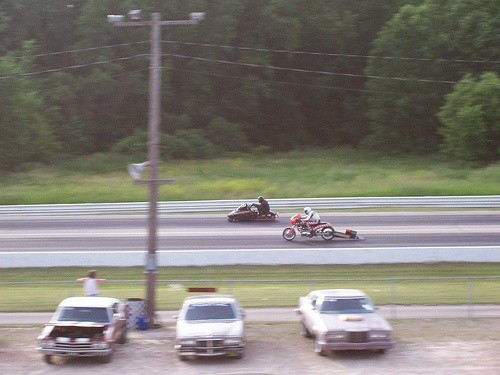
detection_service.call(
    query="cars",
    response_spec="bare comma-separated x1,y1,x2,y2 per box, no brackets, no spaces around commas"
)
35,295,130,364
174,293,247,362
296,288,395,356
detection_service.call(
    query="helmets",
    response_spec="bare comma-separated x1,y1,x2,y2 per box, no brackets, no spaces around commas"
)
258,196,264,203
304,207,312,214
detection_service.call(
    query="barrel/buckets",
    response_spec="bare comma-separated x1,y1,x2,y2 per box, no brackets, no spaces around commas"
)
137,314,149,330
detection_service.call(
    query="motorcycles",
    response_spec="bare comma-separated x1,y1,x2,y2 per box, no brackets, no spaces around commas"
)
282,214,335,241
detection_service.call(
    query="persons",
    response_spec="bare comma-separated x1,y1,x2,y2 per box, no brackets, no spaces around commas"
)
253,196,270,215
301,207,320,230
77,270,107,297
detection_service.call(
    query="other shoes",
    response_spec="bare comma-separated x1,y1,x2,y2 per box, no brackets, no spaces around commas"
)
310,232,315,238
